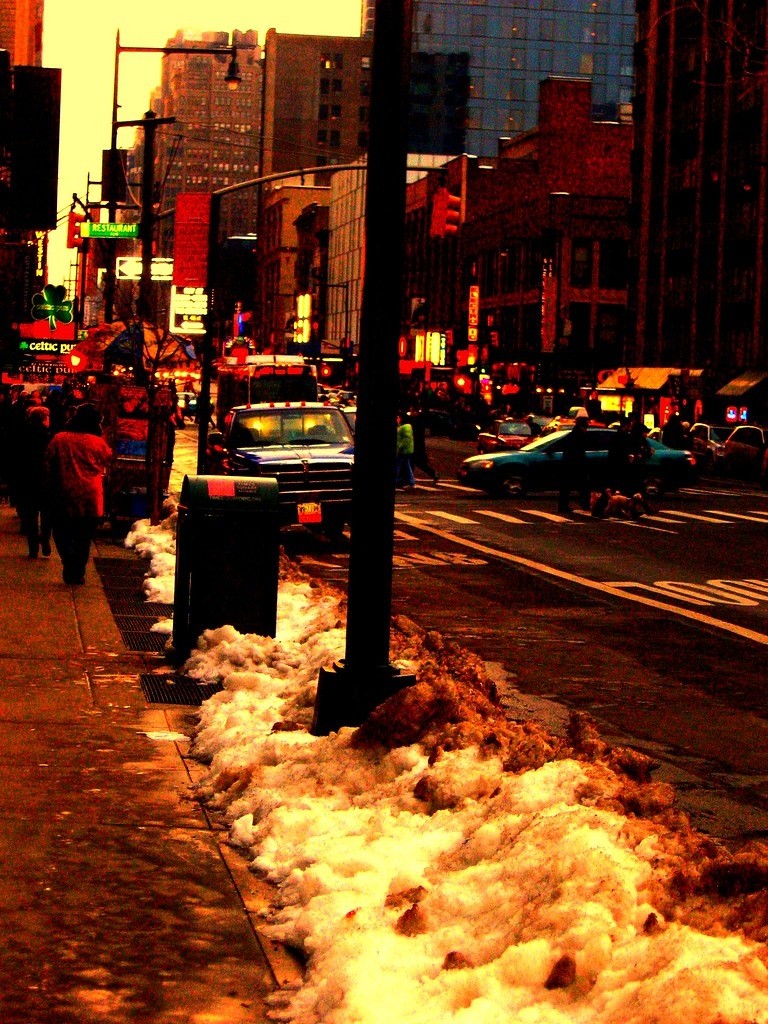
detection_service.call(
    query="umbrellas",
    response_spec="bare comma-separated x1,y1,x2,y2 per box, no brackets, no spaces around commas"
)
60,318,195,373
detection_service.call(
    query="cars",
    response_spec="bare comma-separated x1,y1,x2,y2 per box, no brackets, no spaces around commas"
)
175,392,197,414
686,421,735,471
455,427,697,501
317,382,356,434
723,426,768,476
476,418,538,452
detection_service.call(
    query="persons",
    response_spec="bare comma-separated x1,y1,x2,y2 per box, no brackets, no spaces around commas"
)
341,407,438,493
1,385,75,558
543,415,685,518
41,406,114,585
298,367,317,399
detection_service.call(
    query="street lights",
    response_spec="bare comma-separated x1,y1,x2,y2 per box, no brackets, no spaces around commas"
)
102,31,243,375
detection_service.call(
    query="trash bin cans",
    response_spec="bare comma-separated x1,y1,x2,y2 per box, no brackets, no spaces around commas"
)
172,475,281,650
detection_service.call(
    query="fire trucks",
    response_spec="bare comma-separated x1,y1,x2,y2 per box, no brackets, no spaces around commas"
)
214,353,319,439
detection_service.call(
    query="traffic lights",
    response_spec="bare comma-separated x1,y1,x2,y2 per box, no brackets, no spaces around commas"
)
427,190,462,239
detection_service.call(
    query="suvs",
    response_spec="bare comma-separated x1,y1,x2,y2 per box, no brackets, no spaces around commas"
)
209,400,357,542
